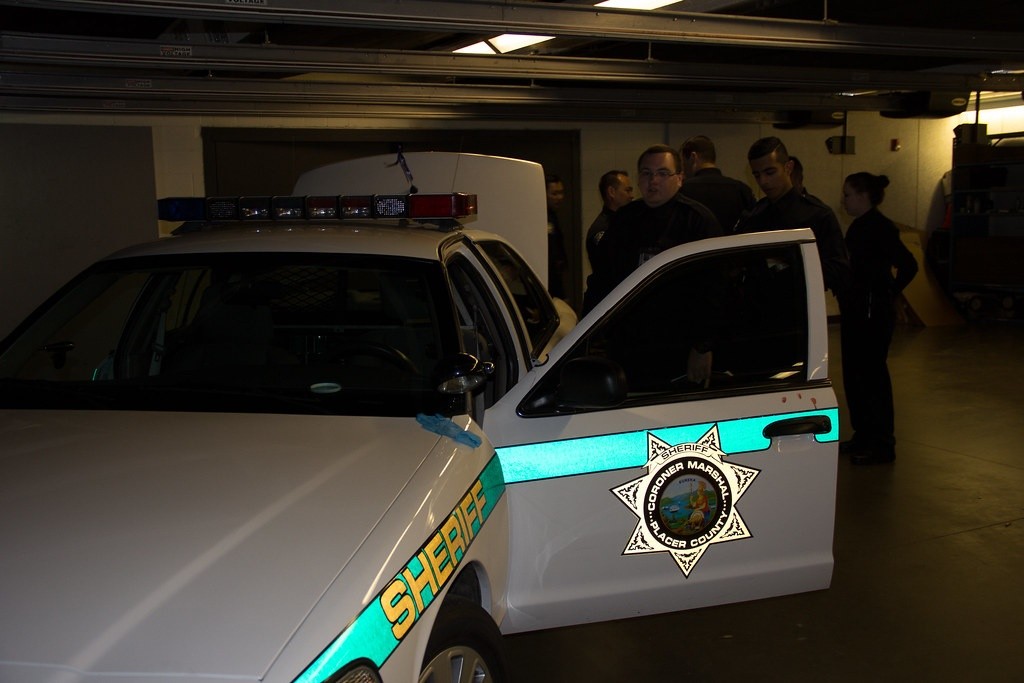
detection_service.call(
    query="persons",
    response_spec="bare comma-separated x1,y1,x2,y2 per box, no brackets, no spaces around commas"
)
576,145,720,401
546,174,567,299
788,156,821,203
730,137,848,375
586,171,633,274
679,134,756,230
837,173,918,461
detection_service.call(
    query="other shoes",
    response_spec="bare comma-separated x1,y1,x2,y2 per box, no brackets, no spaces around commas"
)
840,442,868,464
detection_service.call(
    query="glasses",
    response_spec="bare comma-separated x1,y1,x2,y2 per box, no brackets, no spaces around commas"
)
638,167,676,177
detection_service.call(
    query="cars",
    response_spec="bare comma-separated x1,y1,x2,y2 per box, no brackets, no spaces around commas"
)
923,137,1024,326
0,149,840,683
